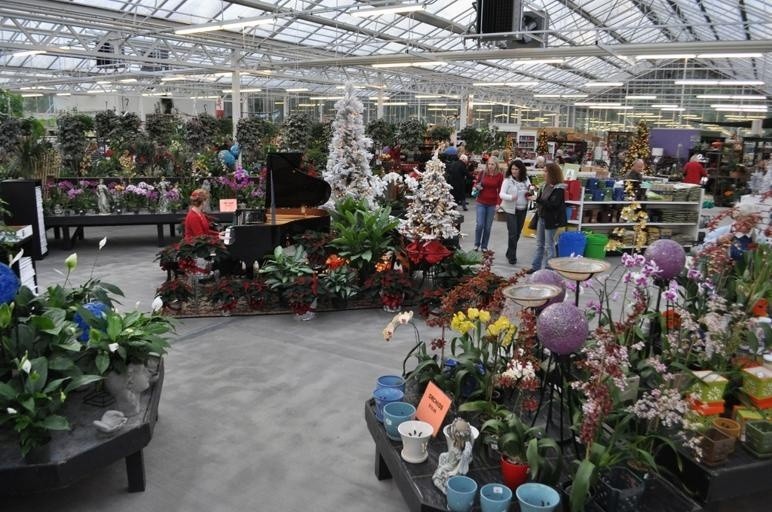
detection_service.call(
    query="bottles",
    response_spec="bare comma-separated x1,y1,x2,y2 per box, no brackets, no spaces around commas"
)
563,176,699,246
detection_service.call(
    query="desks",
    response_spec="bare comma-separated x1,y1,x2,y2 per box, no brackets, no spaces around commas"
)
363,397,605,512
44,210,234,251
0,354,164,494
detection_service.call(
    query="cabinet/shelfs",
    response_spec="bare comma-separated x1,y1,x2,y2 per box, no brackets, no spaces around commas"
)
704,135,772,205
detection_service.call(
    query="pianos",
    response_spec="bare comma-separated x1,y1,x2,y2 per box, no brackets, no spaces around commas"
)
225,153,332,268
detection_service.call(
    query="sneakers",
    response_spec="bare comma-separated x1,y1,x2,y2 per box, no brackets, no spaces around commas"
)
506,251,516,264
526,268,535,274
473,246,479,251
482,247,487,252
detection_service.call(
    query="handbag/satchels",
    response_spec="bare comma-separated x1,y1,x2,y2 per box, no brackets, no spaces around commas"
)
471,182,484,198
497,186,518,215
527,211,538,230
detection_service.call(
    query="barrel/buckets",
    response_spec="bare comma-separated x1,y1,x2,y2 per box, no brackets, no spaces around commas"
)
558,230,586,258
585,233,608,259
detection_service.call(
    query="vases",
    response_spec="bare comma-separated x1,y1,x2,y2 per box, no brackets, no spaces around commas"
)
682,362,772,465
372,375,482,464
378,290,405,314
444,474,561,510
104,363,152,418
18,433,50,465
163,296,318,322
563,176,703,247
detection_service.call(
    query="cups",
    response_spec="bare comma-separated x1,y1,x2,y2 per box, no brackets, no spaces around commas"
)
370,372,561,511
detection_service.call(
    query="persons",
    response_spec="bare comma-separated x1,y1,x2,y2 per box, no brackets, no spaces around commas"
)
185,187,236,278
684,148,710,208
381,141,664,216
498,159,535,267
470,152,504,255
528,162,571,274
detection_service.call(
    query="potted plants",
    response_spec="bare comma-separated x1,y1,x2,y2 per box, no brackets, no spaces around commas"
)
479,411,563,487
329,207,400,288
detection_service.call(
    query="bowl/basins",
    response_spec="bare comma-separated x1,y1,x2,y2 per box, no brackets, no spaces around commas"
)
687,365,772,466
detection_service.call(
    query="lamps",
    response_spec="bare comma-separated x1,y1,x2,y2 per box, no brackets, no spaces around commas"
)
348,2,427,18
169,15,279,32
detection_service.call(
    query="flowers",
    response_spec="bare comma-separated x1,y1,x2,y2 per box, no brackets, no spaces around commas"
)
0,354,73,456
86,296,165,370
2,234,129,361
153,234,451,300
40,166,266,215
